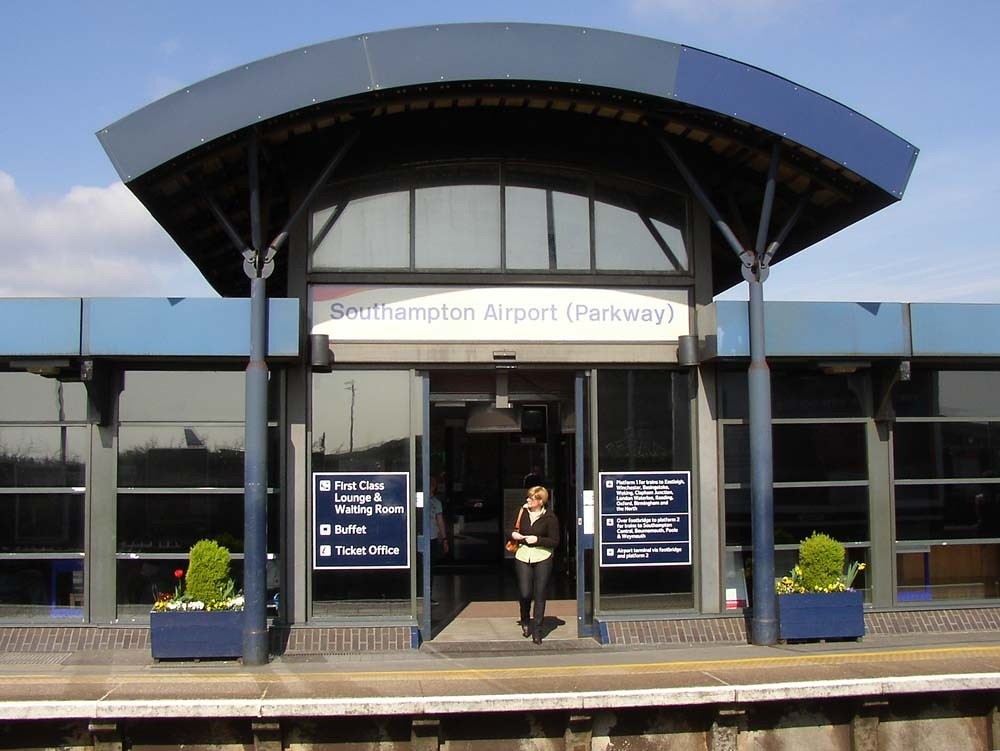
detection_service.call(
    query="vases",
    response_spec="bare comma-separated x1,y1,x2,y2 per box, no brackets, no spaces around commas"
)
778,589,867,642
150,605,244,662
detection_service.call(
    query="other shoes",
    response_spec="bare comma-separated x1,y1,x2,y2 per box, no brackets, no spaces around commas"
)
521,623,532,638
431,600,440,606
533,633,542,645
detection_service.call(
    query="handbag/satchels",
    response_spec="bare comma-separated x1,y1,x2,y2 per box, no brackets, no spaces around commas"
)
505,507,524,554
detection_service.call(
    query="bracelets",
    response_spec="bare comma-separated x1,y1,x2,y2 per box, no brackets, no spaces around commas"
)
442,537,449,540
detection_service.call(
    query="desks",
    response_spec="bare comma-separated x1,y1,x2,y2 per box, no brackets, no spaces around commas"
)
853,545,933,601
51,559,127,605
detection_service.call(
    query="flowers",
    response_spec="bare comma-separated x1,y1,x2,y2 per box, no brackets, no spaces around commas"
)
775,525,868,596
147,539,246,611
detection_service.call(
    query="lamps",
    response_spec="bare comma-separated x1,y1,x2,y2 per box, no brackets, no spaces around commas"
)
306,334,336,368
676,335,700,367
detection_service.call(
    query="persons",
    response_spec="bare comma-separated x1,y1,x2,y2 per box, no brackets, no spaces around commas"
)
430,476,449,606
527,457,570,592
504,486,560,645
